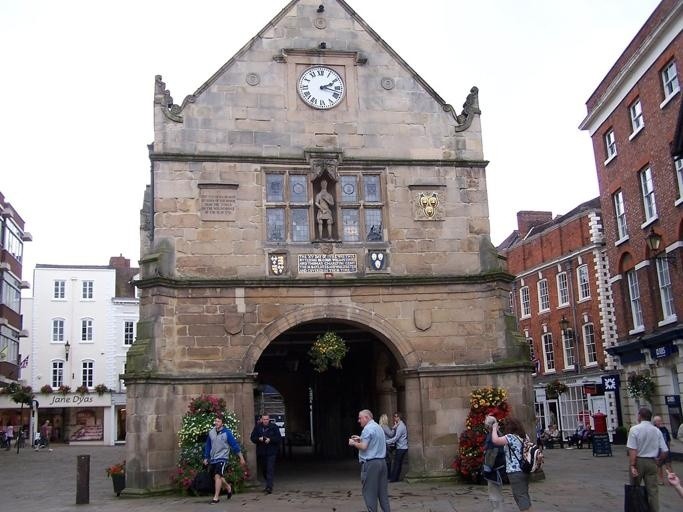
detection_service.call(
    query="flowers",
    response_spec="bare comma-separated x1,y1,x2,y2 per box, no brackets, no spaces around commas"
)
451,384,513,484
103,462,127,477
307,331,351,373
168,394,252,491
627,371,658,403
3,384,109,406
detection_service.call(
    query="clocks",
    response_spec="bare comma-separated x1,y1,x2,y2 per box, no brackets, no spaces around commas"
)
296,65,347,110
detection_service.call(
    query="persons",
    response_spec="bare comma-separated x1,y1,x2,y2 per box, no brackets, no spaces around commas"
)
626,408,669,512
492,417,532,512
349,410,390,512
3,422,14,451
34,420,53,452
666,469,683,498
386,412,408,482
653,416,673,486
250,413,282,494
536,418,591,449
379,414,399,481
482,416,506,512
203,415,245,504
315,180,334,240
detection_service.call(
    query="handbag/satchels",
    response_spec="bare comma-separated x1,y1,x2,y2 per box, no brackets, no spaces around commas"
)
625,485,650,512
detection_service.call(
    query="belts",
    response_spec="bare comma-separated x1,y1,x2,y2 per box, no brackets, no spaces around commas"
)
362,458,384,463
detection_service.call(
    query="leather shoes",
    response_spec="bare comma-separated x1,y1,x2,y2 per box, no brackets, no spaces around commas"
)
264,487,272,494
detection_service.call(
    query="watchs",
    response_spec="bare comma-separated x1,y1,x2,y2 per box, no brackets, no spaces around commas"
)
629,464,636,467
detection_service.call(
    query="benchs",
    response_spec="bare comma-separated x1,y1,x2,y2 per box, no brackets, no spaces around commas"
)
537,430,595,449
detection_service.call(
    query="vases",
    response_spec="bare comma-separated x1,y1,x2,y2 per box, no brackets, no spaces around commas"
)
111,473,124,497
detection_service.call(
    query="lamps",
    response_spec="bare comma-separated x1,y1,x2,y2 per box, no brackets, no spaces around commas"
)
65,340,70,361
558,313,580,340
642,225,677,267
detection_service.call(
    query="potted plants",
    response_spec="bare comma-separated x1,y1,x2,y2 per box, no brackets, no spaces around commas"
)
546,380,569,400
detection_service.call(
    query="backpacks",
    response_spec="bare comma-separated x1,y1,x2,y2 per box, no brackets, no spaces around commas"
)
507,434,544,473
495,443,505,467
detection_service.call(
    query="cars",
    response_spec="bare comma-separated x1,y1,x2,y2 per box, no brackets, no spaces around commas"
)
16,432,42,445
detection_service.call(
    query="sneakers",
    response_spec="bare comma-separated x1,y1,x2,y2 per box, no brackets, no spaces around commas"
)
211,499,220,504
227,485,232,499
